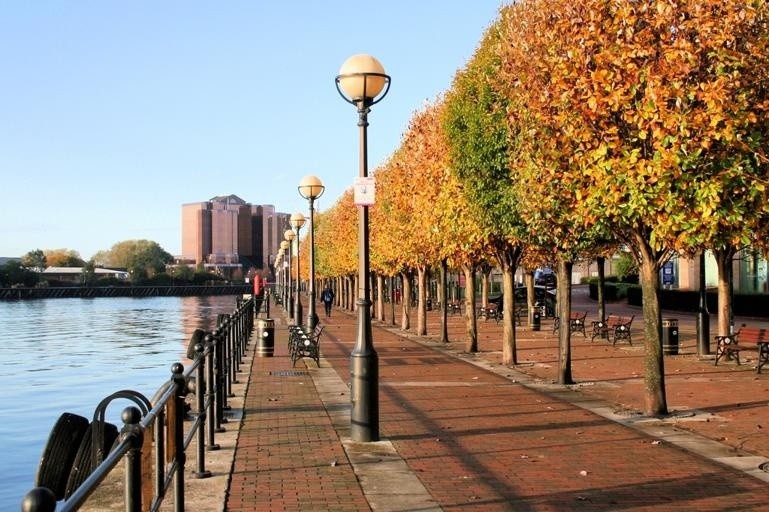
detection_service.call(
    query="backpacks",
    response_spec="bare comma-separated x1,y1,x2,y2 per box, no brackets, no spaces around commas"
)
324,292,332,303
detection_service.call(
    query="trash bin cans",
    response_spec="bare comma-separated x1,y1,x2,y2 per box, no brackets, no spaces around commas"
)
662,318,678,356
425,299,432,311
257,318,274,357
530,306,540,331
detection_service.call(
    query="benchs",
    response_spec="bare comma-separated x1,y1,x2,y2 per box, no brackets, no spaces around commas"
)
550,311,636,348
714,325,769,374
289,321,326,368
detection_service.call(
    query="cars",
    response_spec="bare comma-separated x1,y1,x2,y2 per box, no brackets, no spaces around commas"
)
487,285,557,317
533,266,557,287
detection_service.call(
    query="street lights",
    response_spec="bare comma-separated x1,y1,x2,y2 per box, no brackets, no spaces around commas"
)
328,49,394,444
273,167,324,343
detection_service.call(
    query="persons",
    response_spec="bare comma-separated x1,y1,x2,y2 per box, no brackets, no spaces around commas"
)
320,283,336,317
